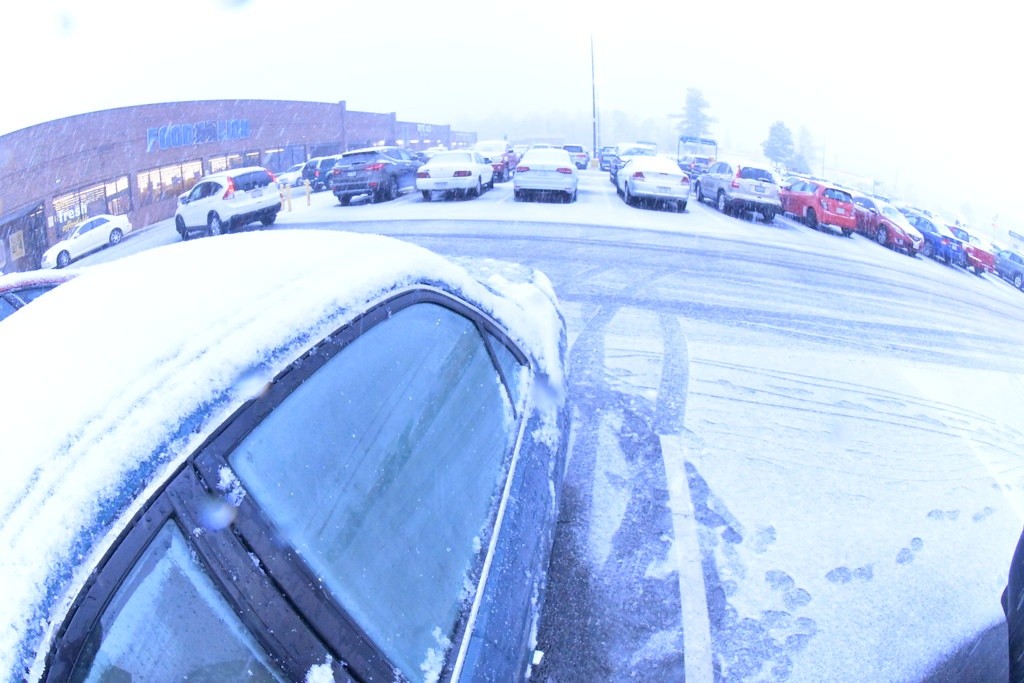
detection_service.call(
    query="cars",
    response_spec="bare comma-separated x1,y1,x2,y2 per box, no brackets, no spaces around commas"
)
853,194,925,255
411,136,889,184
0,224,572,683
947,225,996,276
276,162,308,188
993,248,1024,290
415,150,496,202
780,178,857,235
903,213,963,265
301,155,342,190
41,212,133,270
693,158,782,221
0,268,81,320
614,155,691,211
512,148,580,204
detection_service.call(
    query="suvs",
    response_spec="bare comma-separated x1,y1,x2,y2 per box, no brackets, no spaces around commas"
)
173,165,282,240
331,146,425,205
